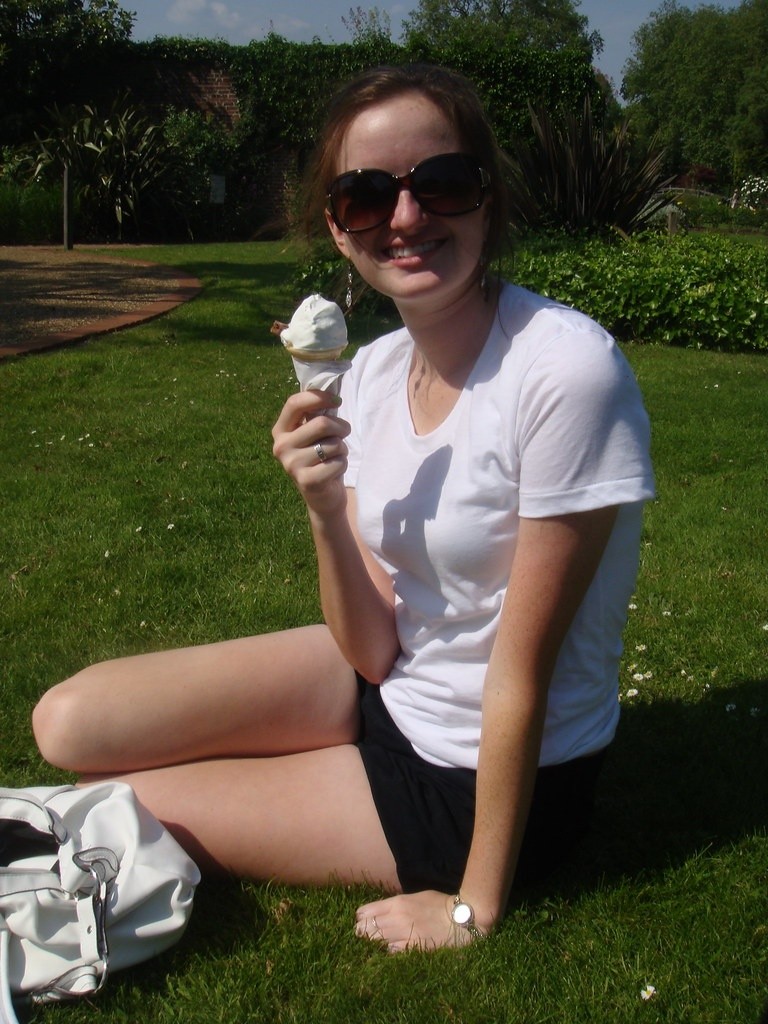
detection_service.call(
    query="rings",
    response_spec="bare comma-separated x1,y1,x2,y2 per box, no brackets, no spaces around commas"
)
372,916,378,928
314,444,326,462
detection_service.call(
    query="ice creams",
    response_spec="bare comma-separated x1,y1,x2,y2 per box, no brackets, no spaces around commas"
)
280,294,348,363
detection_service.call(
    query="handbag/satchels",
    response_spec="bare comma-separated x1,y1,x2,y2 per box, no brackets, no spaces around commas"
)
0,783,201,1022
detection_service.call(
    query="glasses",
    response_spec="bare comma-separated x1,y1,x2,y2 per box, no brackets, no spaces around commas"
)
326,151,491,236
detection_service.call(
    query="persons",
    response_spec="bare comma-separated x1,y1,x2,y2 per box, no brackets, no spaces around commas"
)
33,65,657,952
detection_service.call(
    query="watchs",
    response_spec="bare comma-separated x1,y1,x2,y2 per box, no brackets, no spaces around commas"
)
451,896,480,938
